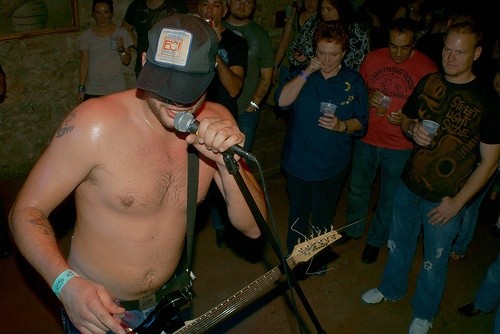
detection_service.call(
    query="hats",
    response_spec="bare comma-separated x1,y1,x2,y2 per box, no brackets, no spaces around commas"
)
135,15,217,105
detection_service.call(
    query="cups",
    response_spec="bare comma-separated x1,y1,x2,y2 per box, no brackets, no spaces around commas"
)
320,102,338,125
376,95,392,116
423,120,439,141
111,36,119,50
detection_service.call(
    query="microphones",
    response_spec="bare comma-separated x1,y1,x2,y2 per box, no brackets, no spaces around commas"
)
174,112,256,162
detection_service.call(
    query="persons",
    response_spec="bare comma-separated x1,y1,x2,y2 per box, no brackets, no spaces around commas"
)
186,0,249,123
78,0,135,103
119,0,189,80
222,0,274,154
9,12,266,334
269,0,500,334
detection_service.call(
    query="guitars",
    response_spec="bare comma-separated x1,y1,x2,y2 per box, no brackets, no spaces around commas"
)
116,224,344,334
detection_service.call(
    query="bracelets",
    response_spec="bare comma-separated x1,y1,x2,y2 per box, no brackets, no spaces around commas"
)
118,45,125,52
343,120,348,133
272,63,278,70
78,85,85,93
299,70,309,79
118,52,125,56
51,269,80,297
250,101,259,109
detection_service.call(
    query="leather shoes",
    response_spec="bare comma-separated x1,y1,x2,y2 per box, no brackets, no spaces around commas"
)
333,231,350,245
362,243,379,264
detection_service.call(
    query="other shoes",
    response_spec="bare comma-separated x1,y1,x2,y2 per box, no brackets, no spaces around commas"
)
216,227,240,248
458,306,494,317
448,253,466,262
313,252,327,276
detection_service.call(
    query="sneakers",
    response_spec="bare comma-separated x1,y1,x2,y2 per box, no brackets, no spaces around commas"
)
361,287,397,304
408,317,432,334
